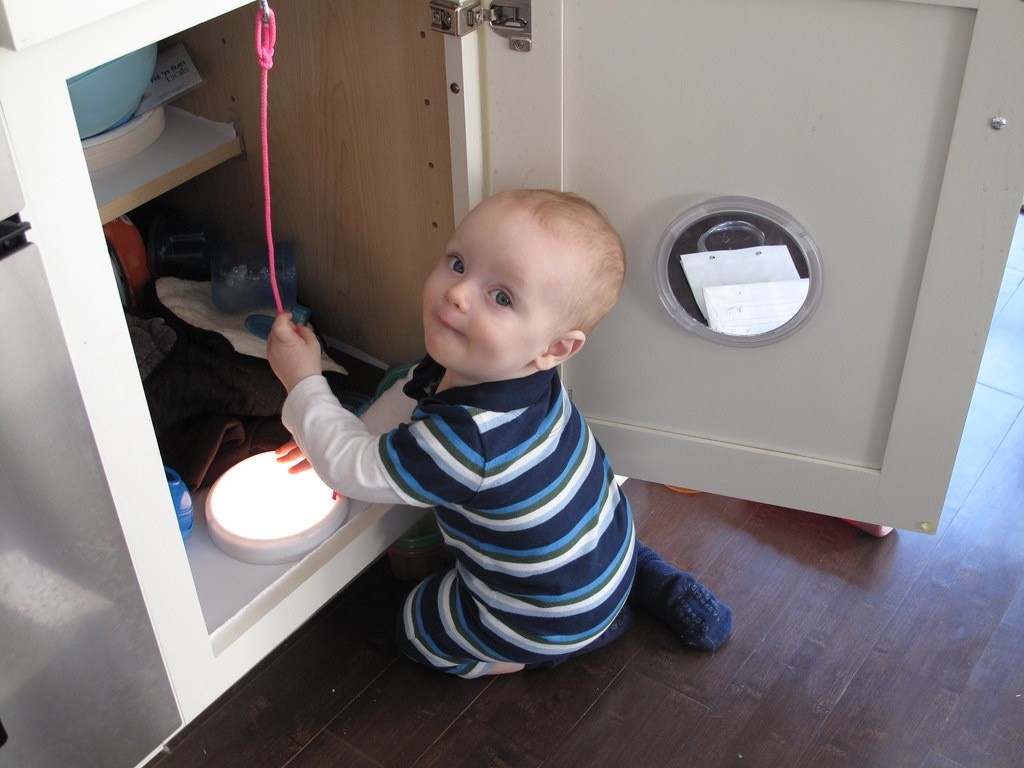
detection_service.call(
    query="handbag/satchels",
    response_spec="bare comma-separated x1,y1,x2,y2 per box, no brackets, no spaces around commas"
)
680,220,810,337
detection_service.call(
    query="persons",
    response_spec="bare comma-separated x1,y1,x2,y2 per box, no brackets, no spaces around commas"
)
267,189,732,679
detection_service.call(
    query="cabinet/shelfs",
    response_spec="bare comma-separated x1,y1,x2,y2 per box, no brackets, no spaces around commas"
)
0,0,1024,768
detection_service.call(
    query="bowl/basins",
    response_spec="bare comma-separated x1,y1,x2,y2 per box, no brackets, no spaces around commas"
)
66,43,157,140
